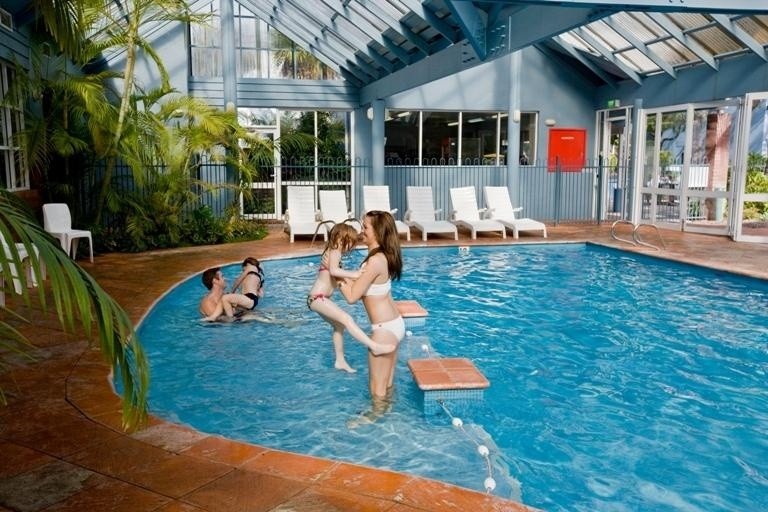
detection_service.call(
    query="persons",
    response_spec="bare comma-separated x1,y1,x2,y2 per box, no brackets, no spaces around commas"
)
200,257,264,323
334,211,405,426
200,268,311,327
307,224,396,374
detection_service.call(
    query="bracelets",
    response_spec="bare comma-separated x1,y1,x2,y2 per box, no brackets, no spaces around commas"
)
337,280,347,289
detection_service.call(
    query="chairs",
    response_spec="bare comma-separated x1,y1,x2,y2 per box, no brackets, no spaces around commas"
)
43,204,93,263
283,186,328,243
319,190,362,235
483,187,546,239
404,187,458,241
363,186,410,241
0,232,40,295
449,187,506,240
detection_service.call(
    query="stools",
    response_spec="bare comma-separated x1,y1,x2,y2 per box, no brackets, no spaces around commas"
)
394,300,428,327
407,357,490,415
